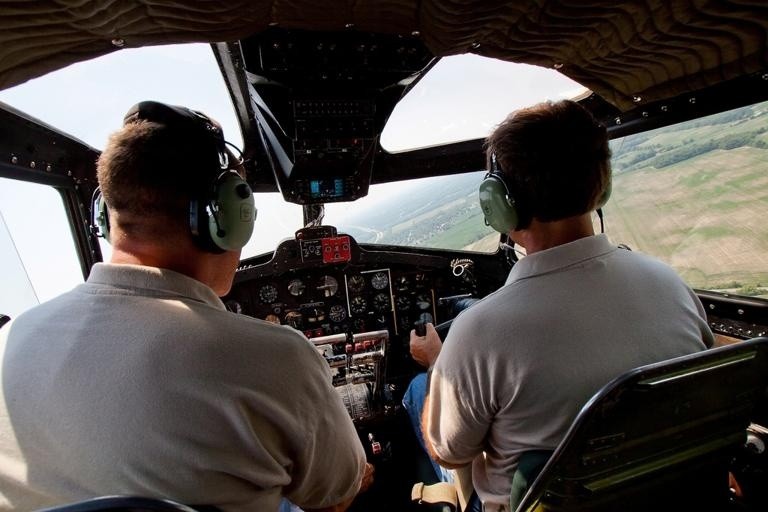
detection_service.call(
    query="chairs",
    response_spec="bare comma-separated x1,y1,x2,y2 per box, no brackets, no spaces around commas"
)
40,491,198,511
509,337,768,508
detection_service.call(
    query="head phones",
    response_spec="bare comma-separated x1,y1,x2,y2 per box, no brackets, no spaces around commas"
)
93,102,254,253
478,145,612,234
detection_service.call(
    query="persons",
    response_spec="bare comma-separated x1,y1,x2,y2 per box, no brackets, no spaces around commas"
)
400,99,715,512
0,100,378,512
